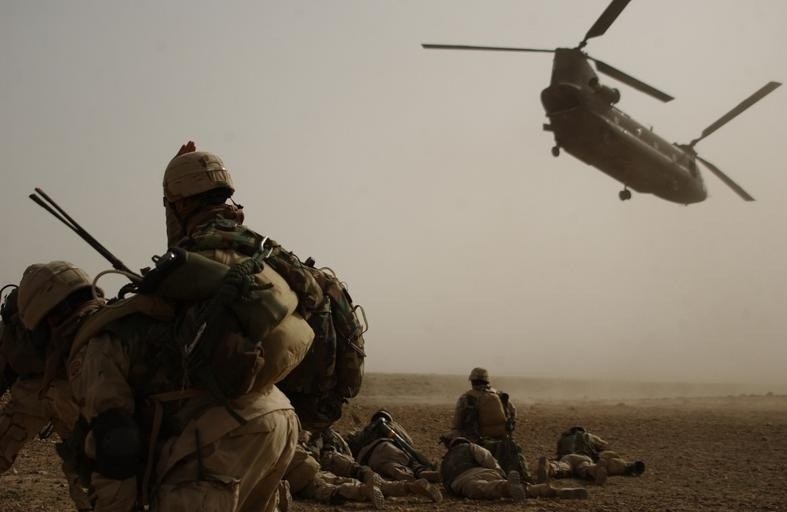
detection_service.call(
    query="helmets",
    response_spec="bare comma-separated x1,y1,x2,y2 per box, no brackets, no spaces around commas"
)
161,151,235,206
469,367,491,383
18,261,104,331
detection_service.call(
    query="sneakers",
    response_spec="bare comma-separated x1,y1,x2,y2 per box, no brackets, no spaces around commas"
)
558,486,589,499
507,469,524,502
417,477,442,502
366,471,385,508
536,457,550,482
593,459,609,486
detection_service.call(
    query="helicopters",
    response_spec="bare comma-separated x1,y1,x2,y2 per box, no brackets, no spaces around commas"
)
421,0,782,208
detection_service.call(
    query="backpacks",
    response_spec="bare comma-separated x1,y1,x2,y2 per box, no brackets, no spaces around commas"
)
139,244,315,399
468,388,508,437
306,265,366,397
